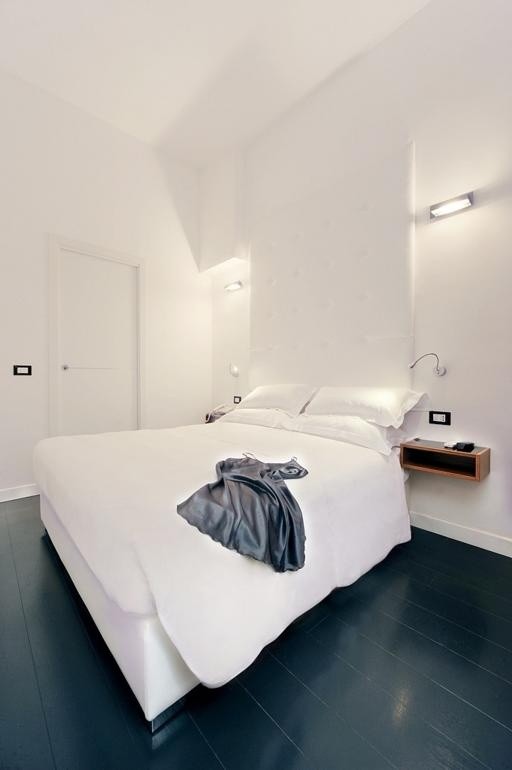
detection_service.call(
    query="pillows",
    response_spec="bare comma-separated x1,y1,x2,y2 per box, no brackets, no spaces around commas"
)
215,384,427,457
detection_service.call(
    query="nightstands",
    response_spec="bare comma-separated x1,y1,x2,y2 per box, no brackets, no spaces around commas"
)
205,414,223,424
400,438,490,481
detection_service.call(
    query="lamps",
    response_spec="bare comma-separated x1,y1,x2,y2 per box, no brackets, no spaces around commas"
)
228,361,239,377
430,191,473,219
223,280,242,292
409,353,446,377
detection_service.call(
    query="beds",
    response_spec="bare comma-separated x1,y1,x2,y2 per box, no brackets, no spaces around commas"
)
30,422,412,733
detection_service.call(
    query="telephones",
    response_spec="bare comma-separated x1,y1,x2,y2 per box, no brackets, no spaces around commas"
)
213,404,233,415
